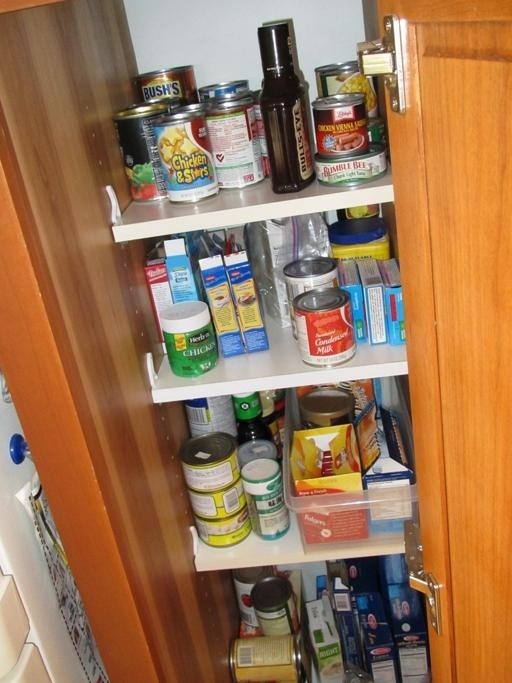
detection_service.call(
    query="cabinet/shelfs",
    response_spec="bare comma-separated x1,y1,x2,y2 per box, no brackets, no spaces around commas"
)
111,163,406,572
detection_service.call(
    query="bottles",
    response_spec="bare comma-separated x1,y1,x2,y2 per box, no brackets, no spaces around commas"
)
254,17,316,196
231,389,274,448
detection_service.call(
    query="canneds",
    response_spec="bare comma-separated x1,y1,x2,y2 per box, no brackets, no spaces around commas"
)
179,431,252,547
230,565,312,683
112,64,271,204
284,257,340,341
312,62,388,188
292,288,356,367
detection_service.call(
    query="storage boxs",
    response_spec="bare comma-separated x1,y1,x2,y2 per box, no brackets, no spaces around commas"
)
283,387,418,555
